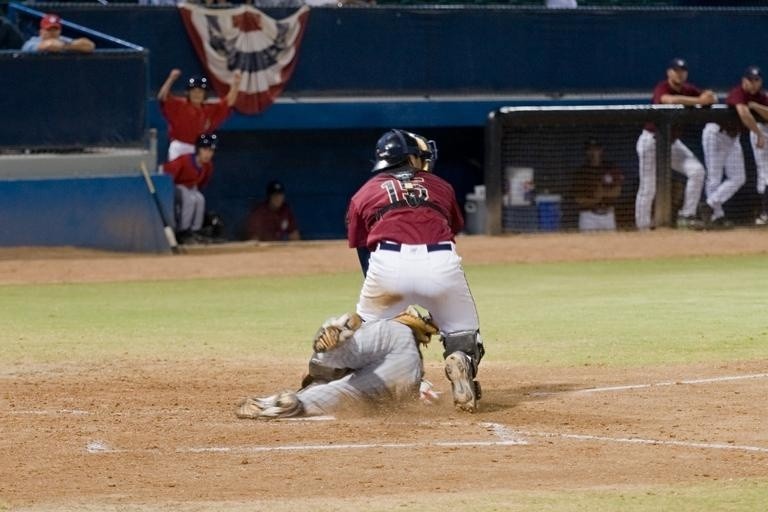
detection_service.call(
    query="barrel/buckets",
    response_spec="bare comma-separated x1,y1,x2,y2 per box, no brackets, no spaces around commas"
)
502,166,535,207
464,192,487,235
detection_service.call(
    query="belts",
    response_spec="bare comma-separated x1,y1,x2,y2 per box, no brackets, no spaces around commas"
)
379,242,451,253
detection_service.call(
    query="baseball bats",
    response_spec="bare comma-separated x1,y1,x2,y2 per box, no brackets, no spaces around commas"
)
137,159,181,257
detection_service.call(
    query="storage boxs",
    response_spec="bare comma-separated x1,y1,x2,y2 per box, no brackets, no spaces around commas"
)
499,198,539,235
464,183,488,236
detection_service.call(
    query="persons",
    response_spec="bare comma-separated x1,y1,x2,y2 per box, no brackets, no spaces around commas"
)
244,181,300,242
573,139,625,230
157,67,242,162
633,58,718,231
158,132,219,244
21,14,96,54
749,89,768,225
313,129,485,416
235,305,440,422
699,65,768,229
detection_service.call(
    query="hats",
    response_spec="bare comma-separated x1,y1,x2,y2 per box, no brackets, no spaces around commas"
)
744,66,762,79
670,58,687,70
41,14,61,30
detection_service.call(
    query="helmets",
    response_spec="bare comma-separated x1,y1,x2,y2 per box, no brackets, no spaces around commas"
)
186,74,209,90
196,132,219,148
371,128,438,172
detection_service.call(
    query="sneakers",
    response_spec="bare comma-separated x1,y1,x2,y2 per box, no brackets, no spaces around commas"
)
313,311,362,352
444,350,477,413
235,392,303,419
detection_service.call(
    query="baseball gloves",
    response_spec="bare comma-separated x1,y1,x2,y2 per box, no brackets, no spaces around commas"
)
396,313,437,342
